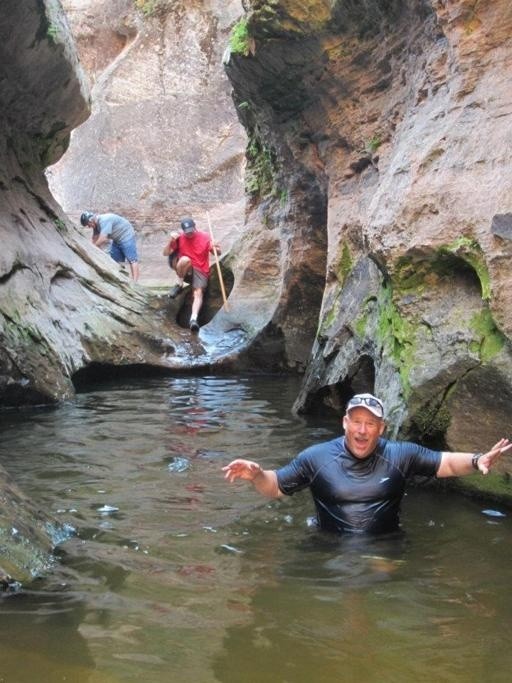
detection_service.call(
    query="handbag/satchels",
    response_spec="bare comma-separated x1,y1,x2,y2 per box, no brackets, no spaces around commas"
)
168,230,179,270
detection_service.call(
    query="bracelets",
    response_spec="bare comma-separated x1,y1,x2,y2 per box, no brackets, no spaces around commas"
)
472,452,483,471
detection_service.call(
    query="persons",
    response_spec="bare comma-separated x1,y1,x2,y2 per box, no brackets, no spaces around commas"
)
81,211,139,283
221,393,512,536
163,218,221,330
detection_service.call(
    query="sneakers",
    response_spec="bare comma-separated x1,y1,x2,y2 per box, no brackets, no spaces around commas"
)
189,320,200,331
170,282,182,299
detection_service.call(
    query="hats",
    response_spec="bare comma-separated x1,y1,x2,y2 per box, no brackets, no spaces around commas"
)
181,218,195,233
346,392,385,421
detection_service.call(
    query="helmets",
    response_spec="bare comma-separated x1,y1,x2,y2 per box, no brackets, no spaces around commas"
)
80,211,93,226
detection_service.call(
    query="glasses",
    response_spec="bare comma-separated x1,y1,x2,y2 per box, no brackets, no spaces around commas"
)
343,397,384,414
